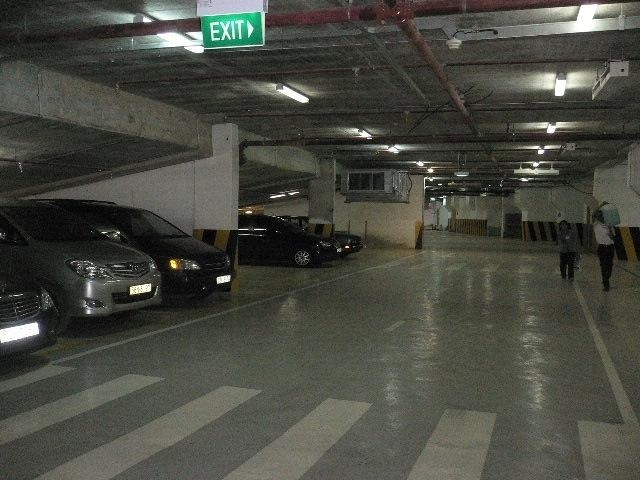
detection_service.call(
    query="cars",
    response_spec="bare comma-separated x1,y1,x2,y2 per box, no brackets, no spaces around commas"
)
0,199,232,356
239,215,363,268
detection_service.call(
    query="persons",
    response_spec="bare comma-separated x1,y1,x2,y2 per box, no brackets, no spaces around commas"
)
556,220,575,281
592,201,616,291
